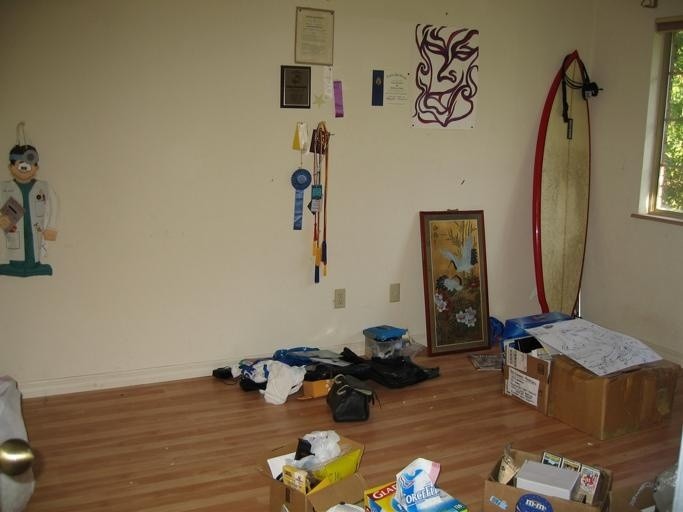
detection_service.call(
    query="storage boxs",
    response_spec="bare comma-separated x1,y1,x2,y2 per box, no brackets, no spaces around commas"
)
483,447,666,512
504,325,680,441
254,434,370,512
363,328,402,359
363,476,468,512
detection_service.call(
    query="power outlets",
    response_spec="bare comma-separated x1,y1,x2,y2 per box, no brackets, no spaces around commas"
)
335,289,346,308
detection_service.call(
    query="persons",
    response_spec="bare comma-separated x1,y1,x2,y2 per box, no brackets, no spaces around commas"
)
0,144,57,277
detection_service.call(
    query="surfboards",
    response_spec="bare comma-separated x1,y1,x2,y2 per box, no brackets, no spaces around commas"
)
531,50,590,316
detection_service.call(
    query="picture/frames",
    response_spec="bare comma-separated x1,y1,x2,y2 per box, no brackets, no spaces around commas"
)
419,209,490,357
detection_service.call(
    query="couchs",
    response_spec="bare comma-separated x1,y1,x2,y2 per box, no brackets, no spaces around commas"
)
0,374,36,512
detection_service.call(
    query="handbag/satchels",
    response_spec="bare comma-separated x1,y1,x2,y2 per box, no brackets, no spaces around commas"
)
326,373,381,422
